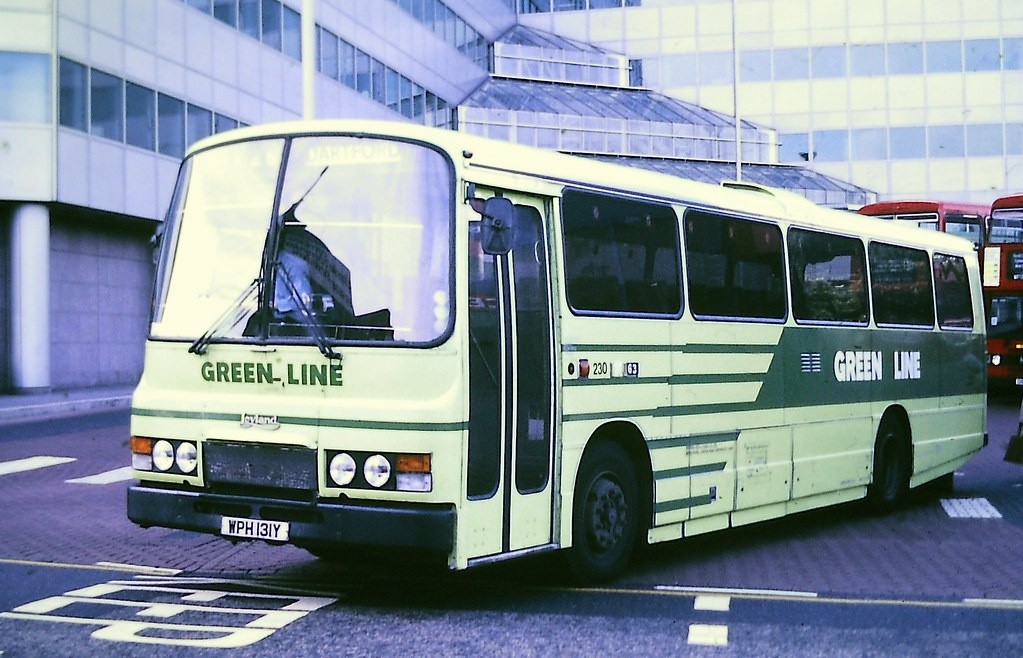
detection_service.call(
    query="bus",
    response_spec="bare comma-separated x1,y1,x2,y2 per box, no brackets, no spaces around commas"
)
975,194,1023,396
849,197,993,304
121,115,991,590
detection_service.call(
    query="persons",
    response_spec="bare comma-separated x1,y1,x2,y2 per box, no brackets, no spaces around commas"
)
271,235,322,318
